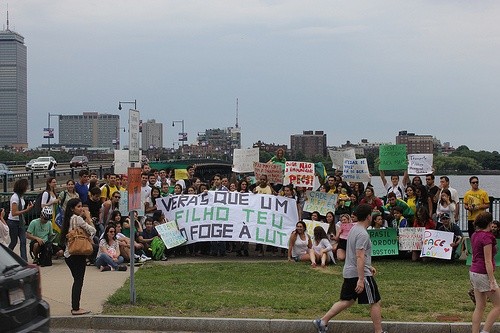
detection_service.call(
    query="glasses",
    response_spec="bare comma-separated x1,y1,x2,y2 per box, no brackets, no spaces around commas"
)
109,231,115,233
114,195,121,199
470,181,479,184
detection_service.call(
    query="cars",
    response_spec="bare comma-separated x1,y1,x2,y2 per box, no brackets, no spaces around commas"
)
0,163,15,182
0,241,51,333
31,156,57,170
25,158,37,172
141,155,149,162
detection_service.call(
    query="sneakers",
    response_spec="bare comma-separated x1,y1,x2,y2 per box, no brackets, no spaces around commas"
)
312,319,329,333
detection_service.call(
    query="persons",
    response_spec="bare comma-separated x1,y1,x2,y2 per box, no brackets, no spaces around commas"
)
266,148,289,168
423,174,439,214
64,198,97,315
311,205,384,333
74,170,90,204
0,206,12,249
183,166,197,189
309,225,336,268
6,178,34,263
144,186,161,216
156,169,171,186
100,173,120,204
468,211,500,333
488,220,500,240
287,221,315,264
321,175,340,195
26,148,467,273
462,176,490,238
357,188,384,208
140,163,151,176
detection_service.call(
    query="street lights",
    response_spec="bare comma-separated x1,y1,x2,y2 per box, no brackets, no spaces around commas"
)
172,120,184,155
117,99,137,110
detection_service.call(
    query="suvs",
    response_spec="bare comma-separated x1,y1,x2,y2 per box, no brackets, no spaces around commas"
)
69,156,88,168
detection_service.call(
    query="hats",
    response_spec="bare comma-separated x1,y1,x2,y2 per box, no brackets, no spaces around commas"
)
440,213,450,220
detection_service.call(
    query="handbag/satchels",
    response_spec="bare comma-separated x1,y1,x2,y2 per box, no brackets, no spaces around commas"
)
468,287,489,305
66,217,93,256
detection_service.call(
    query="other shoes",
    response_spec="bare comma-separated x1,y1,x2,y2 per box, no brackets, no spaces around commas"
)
321,263,325,269
280,251,285,257
118,265,127,271
162,254,167,261
32,257,38,263
310,262,317,267
134,254,152,263
271,251,277,257
172,248,250,257
100,265,105,272
258,249,264,256
70,308,91,315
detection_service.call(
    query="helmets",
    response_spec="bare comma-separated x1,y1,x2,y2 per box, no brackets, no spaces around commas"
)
42,206,53,221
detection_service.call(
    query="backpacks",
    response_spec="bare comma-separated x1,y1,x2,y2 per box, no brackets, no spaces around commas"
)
29,240,52,267
35,190,58,208
